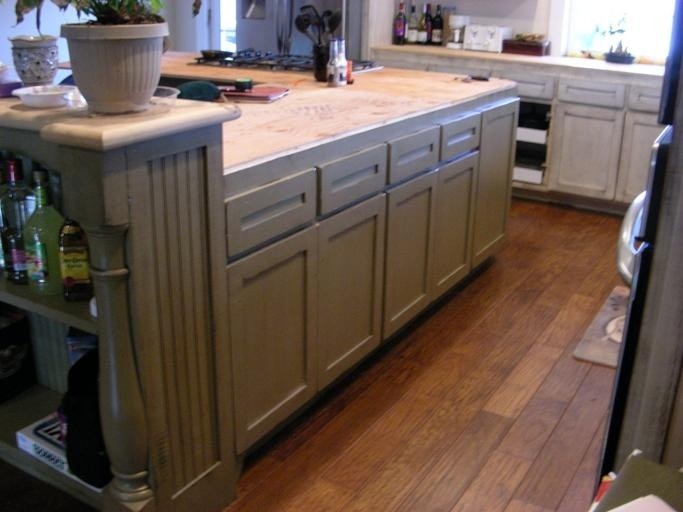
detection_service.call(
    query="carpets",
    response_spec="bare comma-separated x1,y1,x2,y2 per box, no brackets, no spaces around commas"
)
572,284,632,368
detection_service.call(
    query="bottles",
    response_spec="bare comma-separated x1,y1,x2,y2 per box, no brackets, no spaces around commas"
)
419,3,427,43
326,39,337,82
0,158,37,285
24,168,67,298
59,217,94,302
432,5,444,45
334,36,347,85
408,1,418,44
394,1,407,45
427,4,432,43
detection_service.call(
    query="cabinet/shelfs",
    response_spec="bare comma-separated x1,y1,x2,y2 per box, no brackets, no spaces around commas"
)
372,44,559,203
382,107,482,341
224,140,389,457
471,94,522,271
0,95,241,512
548,62,667,216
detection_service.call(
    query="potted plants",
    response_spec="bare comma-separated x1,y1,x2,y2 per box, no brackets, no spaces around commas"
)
14,0,170,115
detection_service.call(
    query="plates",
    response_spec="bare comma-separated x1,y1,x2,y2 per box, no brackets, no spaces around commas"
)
62,86,180,119
11,84,75,108
